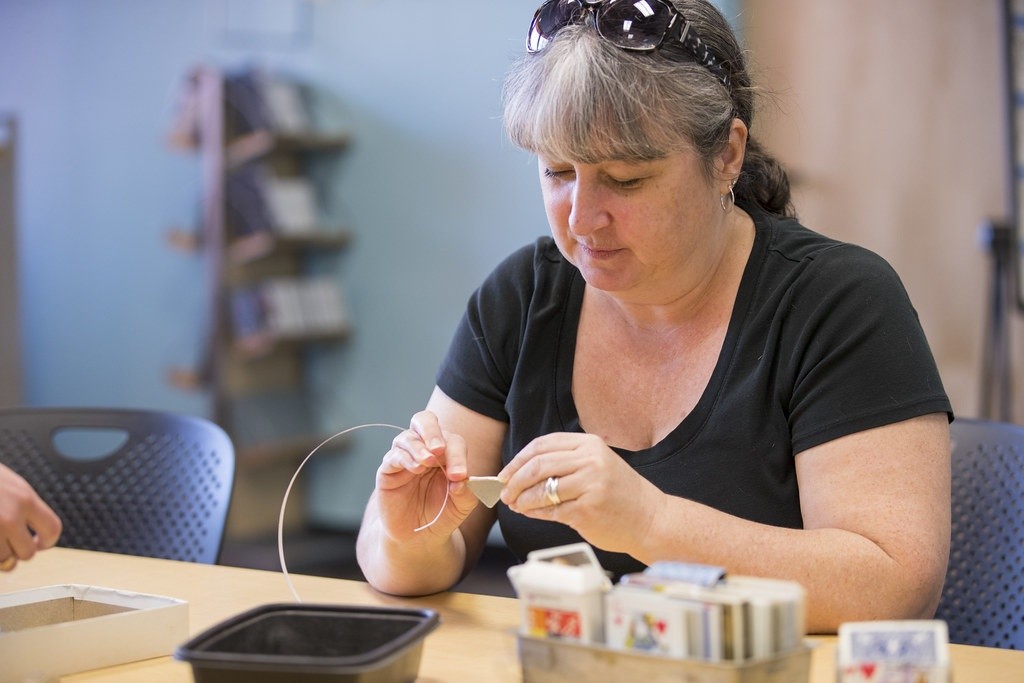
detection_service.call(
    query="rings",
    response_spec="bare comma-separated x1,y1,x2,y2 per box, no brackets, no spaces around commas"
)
0,556,16,570
545,475,562,506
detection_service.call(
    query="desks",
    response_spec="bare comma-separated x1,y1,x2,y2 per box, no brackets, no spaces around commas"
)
0,546,1024,683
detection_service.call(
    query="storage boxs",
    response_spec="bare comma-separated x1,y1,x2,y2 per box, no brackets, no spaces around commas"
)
0,582,190,683
504,622,817,683
174,602,440,683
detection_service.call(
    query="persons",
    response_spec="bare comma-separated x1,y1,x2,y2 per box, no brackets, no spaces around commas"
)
353,0,956,636
0,462,64,574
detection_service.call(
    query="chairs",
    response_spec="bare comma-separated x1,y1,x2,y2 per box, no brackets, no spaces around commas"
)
934,417,1024,650
0,409,237,566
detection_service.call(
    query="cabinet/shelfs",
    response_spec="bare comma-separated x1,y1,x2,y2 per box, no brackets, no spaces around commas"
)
161,64,355,544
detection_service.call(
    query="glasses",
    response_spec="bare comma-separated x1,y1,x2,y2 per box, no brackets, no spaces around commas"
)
525,0,736,98
